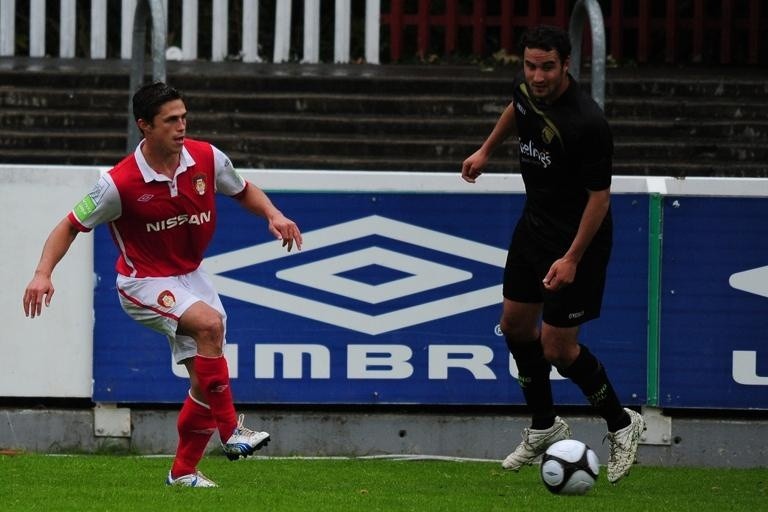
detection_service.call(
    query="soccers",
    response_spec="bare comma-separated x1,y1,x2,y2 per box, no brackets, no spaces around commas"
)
540,439,600,496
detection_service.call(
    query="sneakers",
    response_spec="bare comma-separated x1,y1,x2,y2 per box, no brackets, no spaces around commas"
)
165,469,220,489
606,406,645,484
501,415,574,474
221,424,271,461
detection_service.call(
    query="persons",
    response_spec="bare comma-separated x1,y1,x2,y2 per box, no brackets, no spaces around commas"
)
22,84,303,488
457,23,647,484
